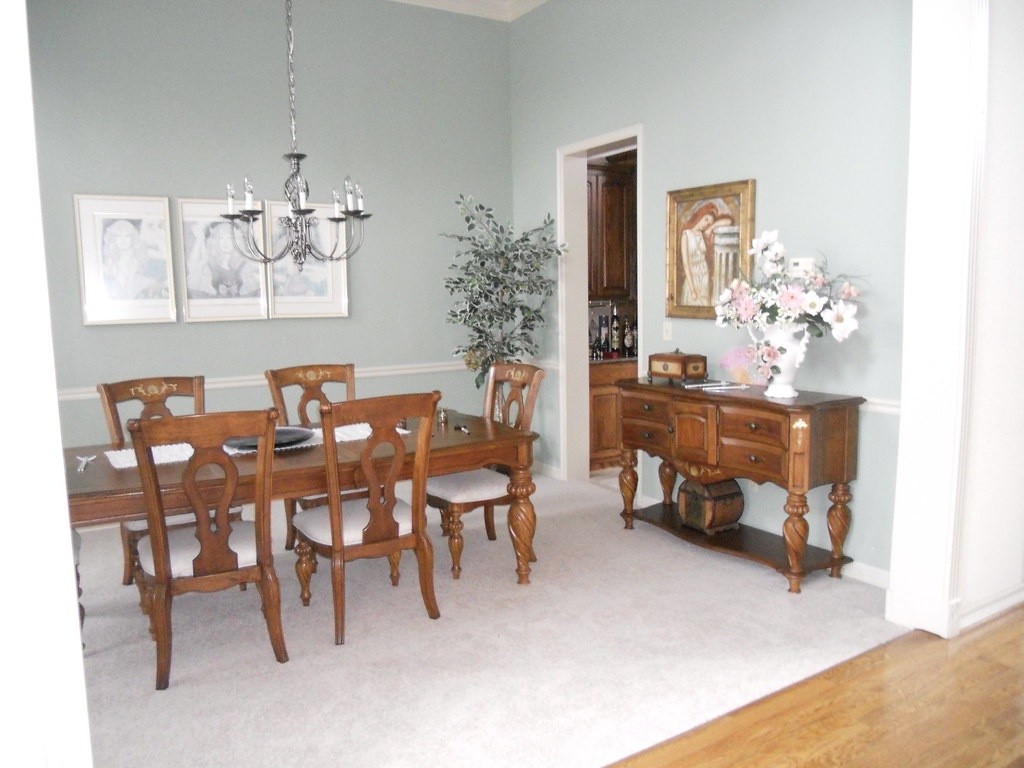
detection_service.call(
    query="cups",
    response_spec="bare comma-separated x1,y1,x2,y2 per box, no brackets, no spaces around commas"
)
436,407,447,424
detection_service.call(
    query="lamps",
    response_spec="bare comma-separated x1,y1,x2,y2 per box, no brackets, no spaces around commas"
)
221,0,374,271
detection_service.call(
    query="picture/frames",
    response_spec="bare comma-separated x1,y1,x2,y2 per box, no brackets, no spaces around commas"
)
266,201,349,320
176,198,270,321
75,192,175,326
667,178,755,322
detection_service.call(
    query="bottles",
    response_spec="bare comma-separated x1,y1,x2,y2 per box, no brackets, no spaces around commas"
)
623,316,638,357
610,306,619,352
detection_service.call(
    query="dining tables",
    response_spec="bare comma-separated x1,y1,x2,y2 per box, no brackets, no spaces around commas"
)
66,407,539,653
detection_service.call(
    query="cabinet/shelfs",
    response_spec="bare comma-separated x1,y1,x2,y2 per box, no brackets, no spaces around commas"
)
614,376,867,593
587,164,637,298
590,360,637,472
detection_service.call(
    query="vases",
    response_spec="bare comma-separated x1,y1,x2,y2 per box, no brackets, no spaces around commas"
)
756,325,808,400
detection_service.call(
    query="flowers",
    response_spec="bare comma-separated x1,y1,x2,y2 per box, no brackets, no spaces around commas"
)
715,230,866,379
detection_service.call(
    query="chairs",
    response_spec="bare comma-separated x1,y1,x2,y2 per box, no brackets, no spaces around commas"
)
94,365,442,690
424,363,544,579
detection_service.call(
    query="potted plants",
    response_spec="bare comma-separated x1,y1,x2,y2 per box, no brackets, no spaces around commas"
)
437,194,570,476
